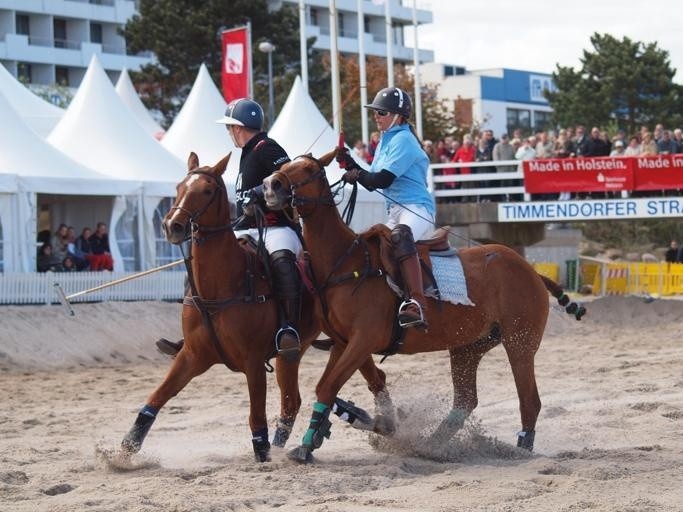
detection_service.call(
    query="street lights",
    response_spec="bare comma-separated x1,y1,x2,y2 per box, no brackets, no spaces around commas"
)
259,42,277,130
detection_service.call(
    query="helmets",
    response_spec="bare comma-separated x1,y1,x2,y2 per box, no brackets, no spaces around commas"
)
361,85,413,120
212,96,266,131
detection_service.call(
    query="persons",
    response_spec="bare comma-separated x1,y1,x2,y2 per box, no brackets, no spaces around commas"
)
333,87,437,334
36,220,112,273
356,119,683,201
664,238,683,264
156,97,304,363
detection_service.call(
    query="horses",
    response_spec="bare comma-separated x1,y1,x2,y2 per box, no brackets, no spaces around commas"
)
260,146,587,463
120,150,395,457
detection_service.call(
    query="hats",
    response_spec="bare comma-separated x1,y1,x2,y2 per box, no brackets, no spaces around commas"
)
421,125,625,153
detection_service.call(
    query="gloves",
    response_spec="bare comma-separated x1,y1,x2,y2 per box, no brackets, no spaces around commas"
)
240,188,259,219
340,167,362,185
335,144,355,171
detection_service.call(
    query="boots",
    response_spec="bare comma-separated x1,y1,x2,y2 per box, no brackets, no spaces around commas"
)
275,294,307,354
154,337,185,358
397,252,430,331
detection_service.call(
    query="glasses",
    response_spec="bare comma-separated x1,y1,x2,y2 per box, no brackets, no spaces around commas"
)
371,107,392,117
